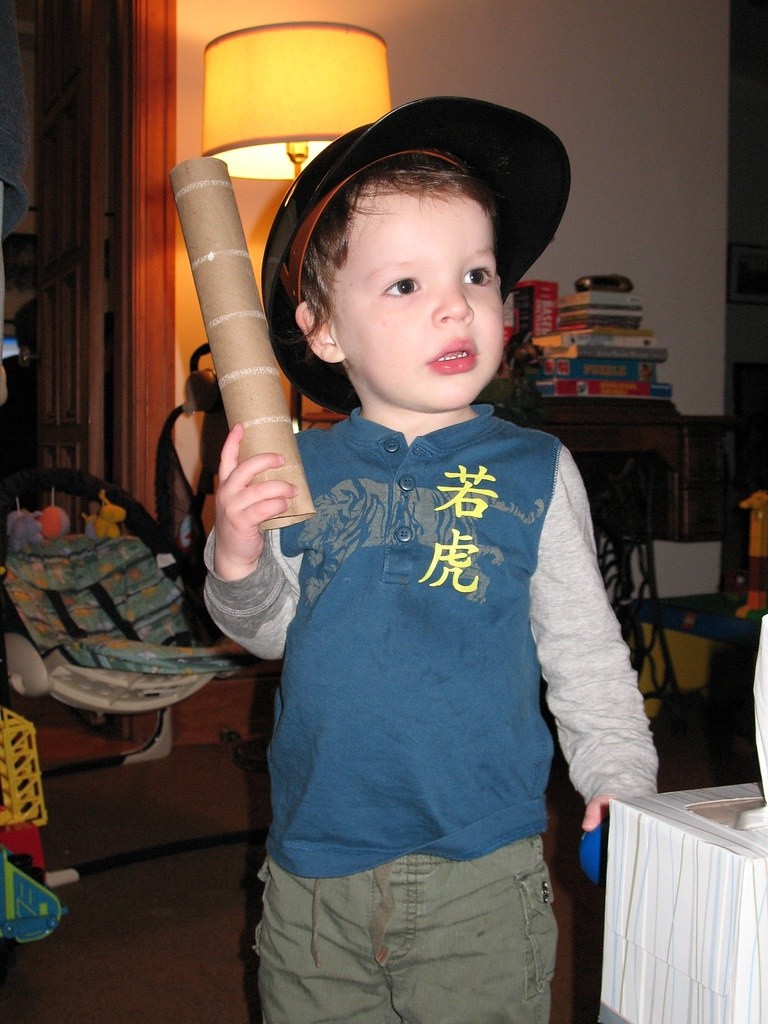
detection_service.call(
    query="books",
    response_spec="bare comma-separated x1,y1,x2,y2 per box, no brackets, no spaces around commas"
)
503,279,672,399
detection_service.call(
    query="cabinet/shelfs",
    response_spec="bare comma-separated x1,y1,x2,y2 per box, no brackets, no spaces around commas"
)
500,396,735,543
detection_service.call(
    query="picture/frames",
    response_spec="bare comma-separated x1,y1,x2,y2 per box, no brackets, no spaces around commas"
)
726,242,768,304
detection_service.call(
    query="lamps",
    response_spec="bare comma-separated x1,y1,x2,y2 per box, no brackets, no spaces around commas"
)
202,20,389,432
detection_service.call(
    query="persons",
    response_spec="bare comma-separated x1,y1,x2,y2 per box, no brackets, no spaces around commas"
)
203,96,661,1024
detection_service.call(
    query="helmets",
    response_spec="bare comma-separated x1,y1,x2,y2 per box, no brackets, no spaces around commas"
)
261,92,566,415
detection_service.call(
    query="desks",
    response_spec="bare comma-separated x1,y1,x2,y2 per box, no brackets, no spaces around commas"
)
633,591,766,784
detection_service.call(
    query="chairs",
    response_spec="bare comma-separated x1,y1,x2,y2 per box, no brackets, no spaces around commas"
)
0,467,254,888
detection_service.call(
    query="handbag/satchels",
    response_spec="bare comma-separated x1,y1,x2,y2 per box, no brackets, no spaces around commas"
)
0,536,246,672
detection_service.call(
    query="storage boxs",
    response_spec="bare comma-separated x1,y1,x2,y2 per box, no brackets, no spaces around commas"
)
512,280,558,335
